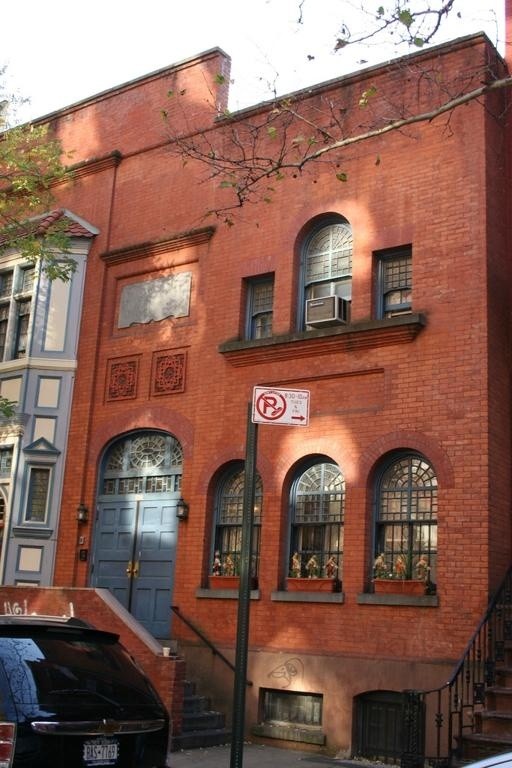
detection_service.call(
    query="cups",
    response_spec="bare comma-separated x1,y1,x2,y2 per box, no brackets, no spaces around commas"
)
162,647,170,657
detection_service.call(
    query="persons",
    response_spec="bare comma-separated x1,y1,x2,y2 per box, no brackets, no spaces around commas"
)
322,555,337,578
305,553,320,578
291,551,302,578
212,550,221,577
372,553,386,579
415,554,431,581
223,553,234,576
391,554,406,578
234,554,240,576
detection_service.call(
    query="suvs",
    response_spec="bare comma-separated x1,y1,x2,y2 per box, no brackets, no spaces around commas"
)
1,616,170,768
